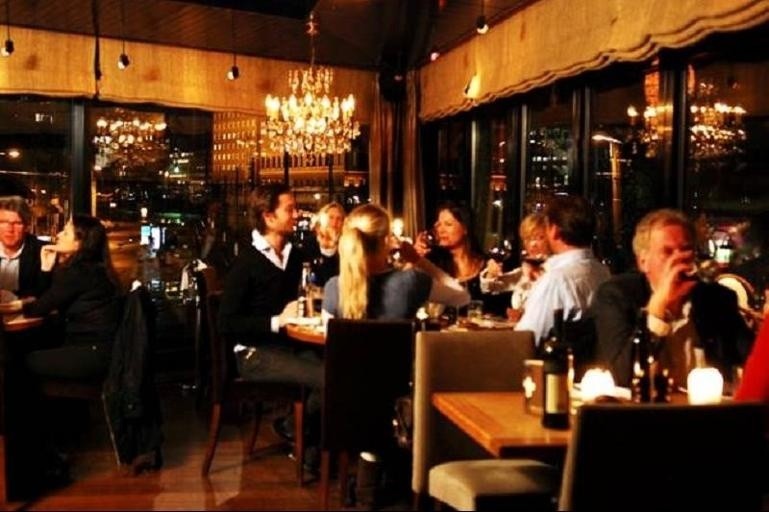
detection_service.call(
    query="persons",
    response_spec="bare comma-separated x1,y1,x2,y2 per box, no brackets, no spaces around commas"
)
218,181,610,487
0,196,123,493
592,208,756,387
733,314,768,402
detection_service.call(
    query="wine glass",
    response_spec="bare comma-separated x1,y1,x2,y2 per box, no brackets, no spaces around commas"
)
413,228,440,252
485,232,517,289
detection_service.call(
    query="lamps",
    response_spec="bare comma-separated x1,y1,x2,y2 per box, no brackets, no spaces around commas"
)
5,1,13,52
265,11,361,156
117,1,129,69
227,9,239,79
475,1,490,34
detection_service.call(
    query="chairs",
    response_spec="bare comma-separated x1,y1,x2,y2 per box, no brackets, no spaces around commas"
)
36,280,154,470
557,400,768,512
194,267,317,488
318,319,443,510
410,328,559,512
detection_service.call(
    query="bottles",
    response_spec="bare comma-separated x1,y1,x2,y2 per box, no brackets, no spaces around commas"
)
542,308,571,431
630,310,651,379
261,152,341,168
628,372,674,403
299,178,330,187
296,261,314,319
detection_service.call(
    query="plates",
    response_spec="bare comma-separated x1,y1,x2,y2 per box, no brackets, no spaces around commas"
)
568,379,631,406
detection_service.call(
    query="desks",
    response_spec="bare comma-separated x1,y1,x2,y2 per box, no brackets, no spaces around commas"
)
0,306,58,511
429,386,735,461
282,312,517,347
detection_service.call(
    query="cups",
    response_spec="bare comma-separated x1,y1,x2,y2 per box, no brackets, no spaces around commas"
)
675,257,699,281
388,232,400,256
520,236,549,266
429,300,445,319
522,358,543,418
467,300,482,321
